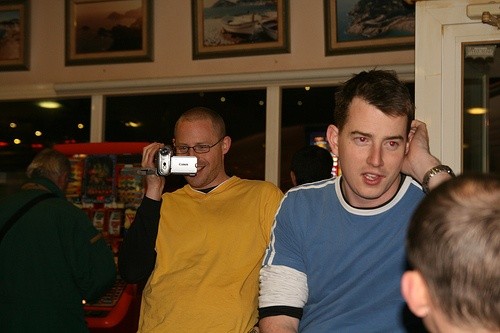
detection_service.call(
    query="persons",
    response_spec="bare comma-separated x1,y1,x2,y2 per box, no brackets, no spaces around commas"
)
117,107,285,333
258,69,456,333
0,148,117,333
400,173,500,333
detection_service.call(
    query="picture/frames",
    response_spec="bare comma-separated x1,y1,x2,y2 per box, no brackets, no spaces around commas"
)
323,0,416,57
190,0,291,62
64,0,155,66
0,0,31,73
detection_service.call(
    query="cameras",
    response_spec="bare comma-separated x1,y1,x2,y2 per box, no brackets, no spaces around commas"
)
156,146,197,177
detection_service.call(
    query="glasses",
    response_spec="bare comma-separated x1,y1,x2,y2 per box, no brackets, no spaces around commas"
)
172,136,225,154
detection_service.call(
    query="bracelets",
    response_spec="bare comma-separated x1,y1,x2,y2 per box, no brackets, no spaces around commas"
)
421,165,455,194
